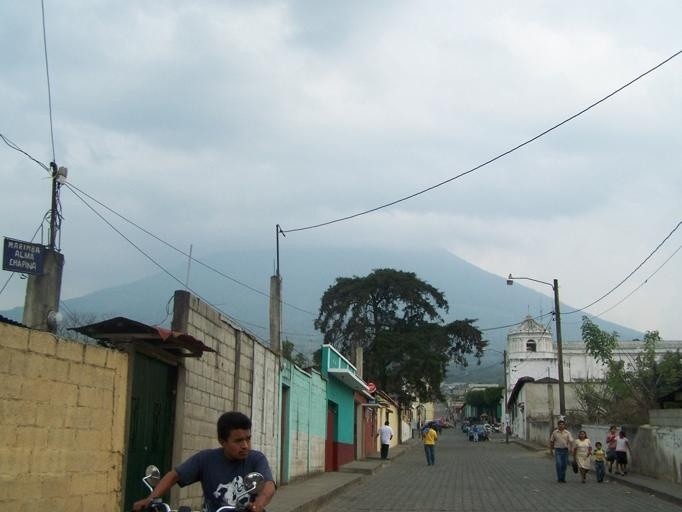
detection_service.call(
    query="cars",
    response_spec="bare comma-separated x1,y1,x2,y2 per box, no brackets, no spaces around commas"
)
461,421,491,442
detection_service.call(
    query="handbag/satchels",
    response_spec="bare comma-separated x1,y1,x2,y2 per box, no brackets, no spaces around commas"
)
571,460,578,473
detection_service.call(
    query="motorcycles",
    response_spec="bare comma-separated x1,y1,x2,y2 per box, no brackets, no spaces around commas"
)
142,465,268,512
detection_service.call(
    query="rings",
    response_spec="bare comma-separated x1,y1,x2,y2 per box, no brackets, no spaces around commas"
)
252,505,257,509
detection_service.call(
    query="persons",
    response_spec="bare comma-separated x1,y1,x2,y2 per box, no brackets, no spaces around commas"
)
590,440,606,483
571,430,593,484
373,421,394,460
604,425,618,472
549,420,574,483
133,410,277,511
421,421,439,466
613,430,632,476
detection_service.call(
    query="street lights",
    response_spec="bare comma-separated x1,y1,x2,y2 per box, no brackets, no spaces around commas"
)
482,348,509,443
507,274,565,416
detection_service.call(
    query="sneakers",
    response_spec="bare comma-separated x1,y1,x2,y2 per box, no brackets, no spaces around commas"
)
581,477,604,483
558,479,566,483
607,466,626,476
